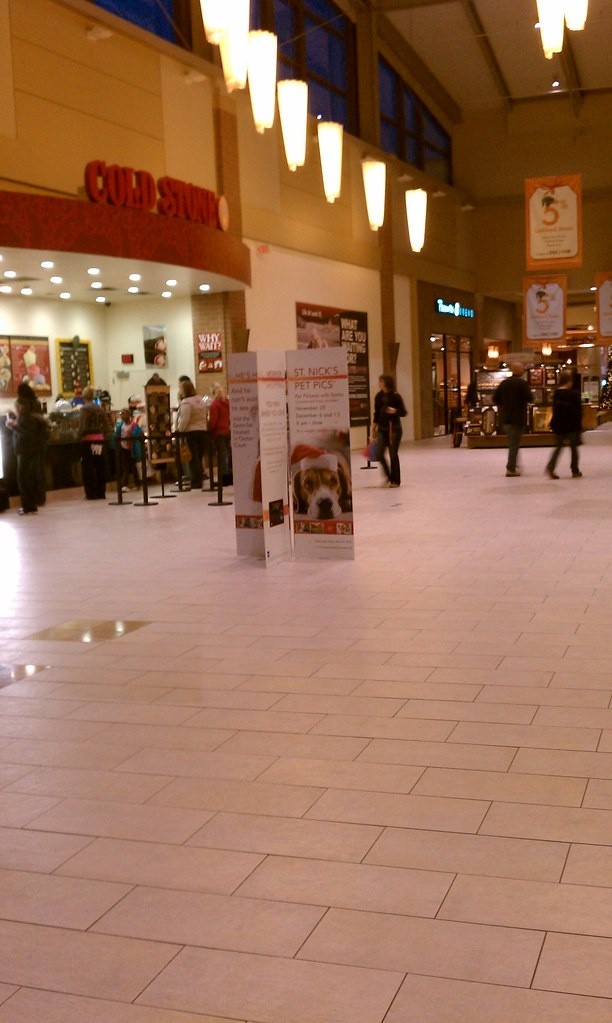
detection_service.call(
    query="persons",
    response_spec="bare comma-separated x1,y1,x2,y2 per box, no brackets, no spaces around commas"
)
492,362,535,477
544,372,584,479
370,374,409,488
169,377,210,488
6,383,50,515
207,382,233,487
112,409,145,492
80,387,108,500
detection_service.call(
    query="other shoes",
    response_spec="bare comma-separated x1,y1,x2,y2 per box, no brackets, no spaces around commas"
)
175,481,191,485
382,478,389,486
506,470,520,477
572,472,583,478
544,468,559,479
389,483,400,488
213,481,233,486
17,508,38,516
122,486,130,493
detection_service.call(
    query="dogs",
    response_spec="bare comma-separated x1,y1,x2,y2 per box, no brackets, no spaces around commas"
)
289,444,351,520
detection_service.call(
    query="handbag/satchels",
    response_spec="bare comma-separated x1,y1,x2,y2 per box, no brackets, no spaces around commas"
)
367,438,378,462
179,437,192,465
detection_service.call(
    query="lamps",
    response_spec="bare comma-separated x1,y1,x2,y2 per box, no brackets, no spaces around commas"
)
199,0,429,253
535,0,588,59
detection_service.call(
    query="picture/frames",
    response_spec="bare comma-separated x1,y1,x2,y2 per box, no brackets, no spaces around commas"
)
465,365,557,436
